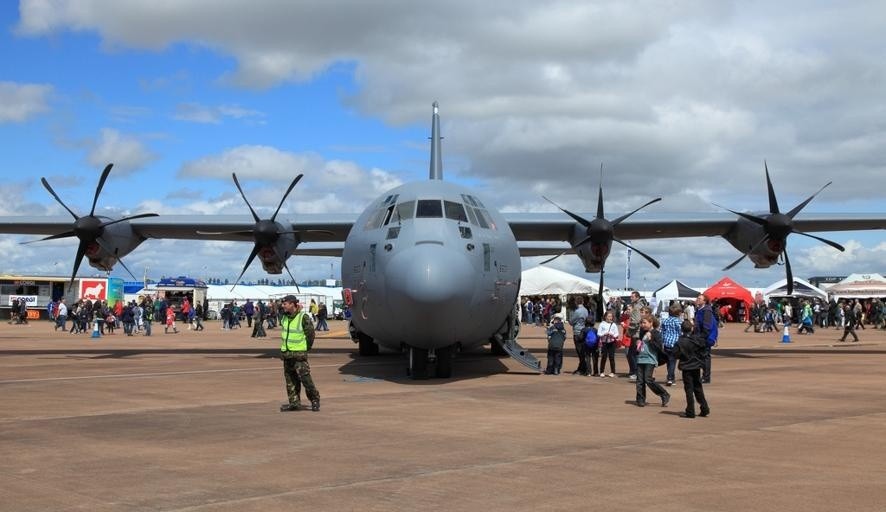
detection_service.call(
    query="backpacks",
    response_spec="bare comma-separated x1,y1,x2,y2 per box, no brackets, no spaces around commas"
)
527,303,532,312
550,329,564,351
585,327,597,347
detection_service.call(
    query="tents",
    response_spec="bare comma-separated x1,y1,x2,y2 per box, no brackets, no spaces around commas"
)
515,265,606,323
762,277,828,310
825,273,886,302
702,279,755,324
651,281,702,313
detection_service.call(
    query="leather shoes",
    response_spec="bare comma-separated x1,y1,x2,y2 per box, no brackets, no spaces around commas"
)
281,404,301,411
545,367,552,374
312,402,319,411
554,368,559,374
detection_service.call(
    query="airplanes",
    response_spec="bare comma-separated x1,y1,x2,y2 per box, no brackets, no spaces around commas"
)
0,101,885,381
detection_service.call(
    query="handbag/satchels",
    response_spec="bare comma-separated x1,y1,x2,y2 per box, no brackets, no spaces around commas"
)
601,321,613,353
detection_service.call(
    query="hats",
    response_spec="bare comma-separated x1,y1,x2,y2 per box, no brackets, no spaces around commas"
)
281,295,299,303
623,304,633,310
551,317,562,324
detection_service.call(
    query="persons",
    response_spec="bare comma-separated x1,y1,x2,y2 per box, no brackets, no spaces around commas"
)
220,304,232,329
544,299,551,325
314,302,329,331
268,300,275,326
597,310,619,378
671,320,710,418
569,296,589,376
280,295,320,412
159,298,167,324
639,306,652,320
94,302,104,335
179,296,190,324
69,304,80,336
827,302,835,326
639,306,652,339
576,317,600,377
243,298,254,327
544,316,566,375
744,303,760,333
53,296,69,332
524,297,533,326
814,302,819,325
153,298,159,322
7,300,20,325
839,305,859,342
635,314,672,407
77,305,88,334
819,300,827,328
875,298,885,329
141,303,152,336
620,302,633,334
783,301,792,328
538,298,544,325
308,298,318,322
853,299,866,330
776,301,783,325
18,299,28,324
105,311,116,335
550,297,557,321
164,302,180,334
869,299,876,323
194,300,204,331
626,291,649,380
186,302,197,330
123,302,134,336
694,294,719,384
46,297,57,322
798,302,814,335
251,307,263,338
131,303,141,334
659,303,683,386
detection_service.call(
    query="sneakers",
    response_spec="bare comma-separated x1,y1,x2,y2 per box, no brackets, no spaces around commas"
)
680,413,688,417
663,396,671,407
672,380,676,387
630,375,638,381
609,373,615,378
593,373,598,376
587,374,591,377
667,378,673,386
600,373,605,377
701,378,710,383
699,413,709,416
573,370,585,375
637,401,645,407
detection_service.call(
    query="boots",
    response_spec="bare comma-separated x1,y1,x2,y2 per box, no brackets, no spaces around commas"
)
165,327,168,334
187,324,191,330
193,323,197,329
173,327,180,334
